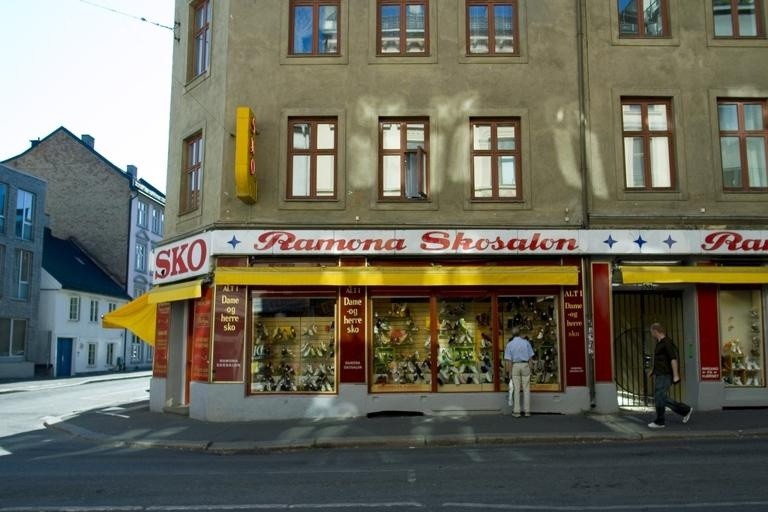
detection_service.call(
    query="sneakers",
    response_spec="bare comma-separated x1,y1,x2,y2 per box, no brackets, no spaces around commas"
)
682,408,693,423
524,412,531,417
498,301,557,384
721,309,761,387
373,304,431,384
252,320,334,391
436,302,493,385
512,412,521,418
648,422,665,428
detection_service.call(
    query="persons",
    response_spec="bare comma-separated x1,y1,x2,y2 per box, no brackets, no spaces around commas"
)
645,322,694,429
505,327,535,419
